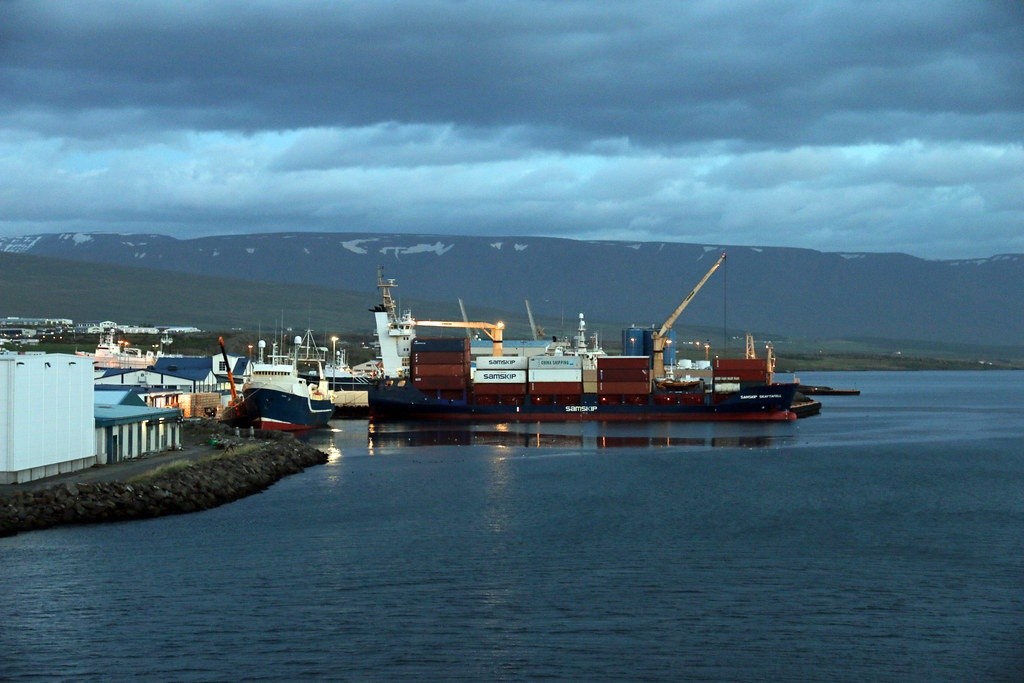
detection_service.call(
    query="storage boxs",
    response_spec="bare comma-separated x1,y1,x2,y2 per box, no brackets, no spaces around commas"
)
411,335,775,400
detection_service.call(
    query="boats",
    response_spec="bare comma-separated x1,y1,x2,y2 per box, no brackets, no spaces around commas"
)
307,365,383,418
368,251,822,421
324,349,353,377
75,329,256,410
242,358,336,432
744,333,861,396
352,329,383,379
